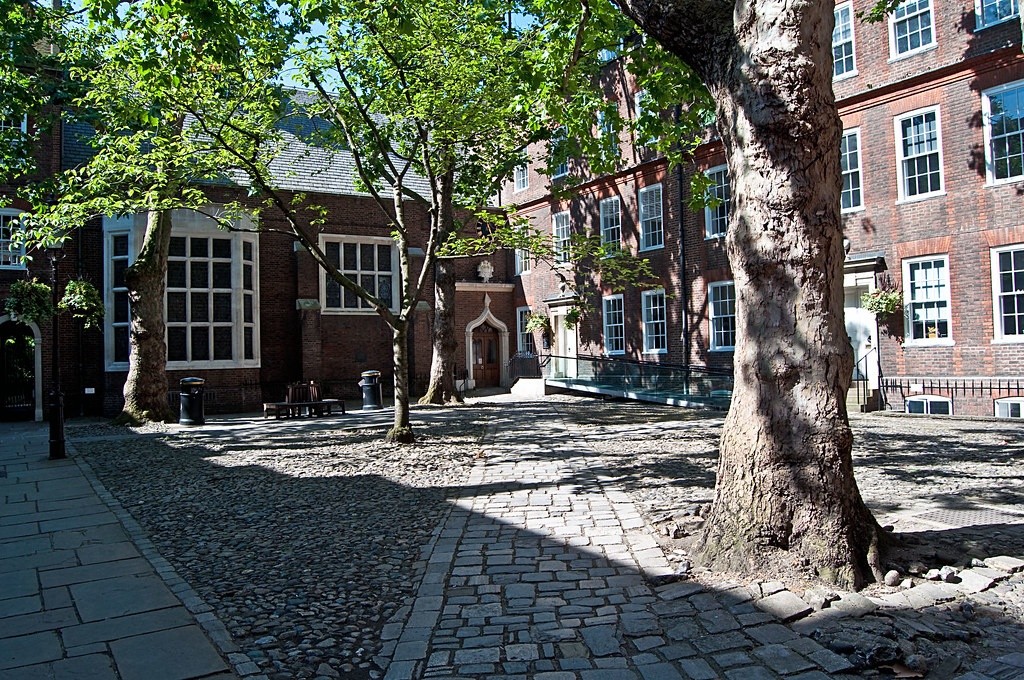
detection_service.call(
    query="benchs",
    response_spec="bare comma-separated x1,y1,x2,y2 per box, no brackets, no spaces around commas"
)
262,382,345,419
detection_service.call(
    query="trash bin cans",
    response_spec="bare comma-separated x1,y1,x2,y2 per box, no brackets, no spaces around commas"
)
178,376,206,426
357,369,385,411
